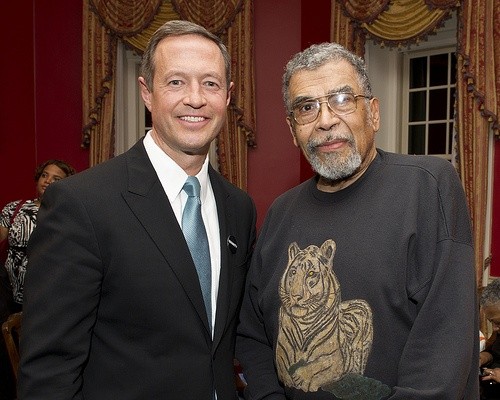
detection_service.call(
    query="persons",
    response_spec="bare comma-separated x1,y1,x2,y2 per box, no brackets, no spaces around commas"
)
480,279,500,384
0,161,75,311
19,20,256,400
233,41,480,400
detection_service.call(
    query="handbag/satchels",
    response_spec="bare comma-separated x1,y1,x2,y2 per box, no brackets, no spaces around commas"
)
0,199,29,265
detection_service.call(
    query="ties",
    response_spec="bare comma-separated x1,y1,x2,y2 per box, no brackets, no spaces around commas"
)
181,176,212,341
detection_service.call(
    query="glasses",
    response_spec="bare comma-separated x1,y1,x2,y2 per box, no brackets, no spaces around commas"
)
288,93,371,125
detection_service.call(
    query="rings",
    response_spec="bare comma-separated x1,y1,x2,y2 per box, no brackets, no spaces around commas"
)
490,371,493,374
